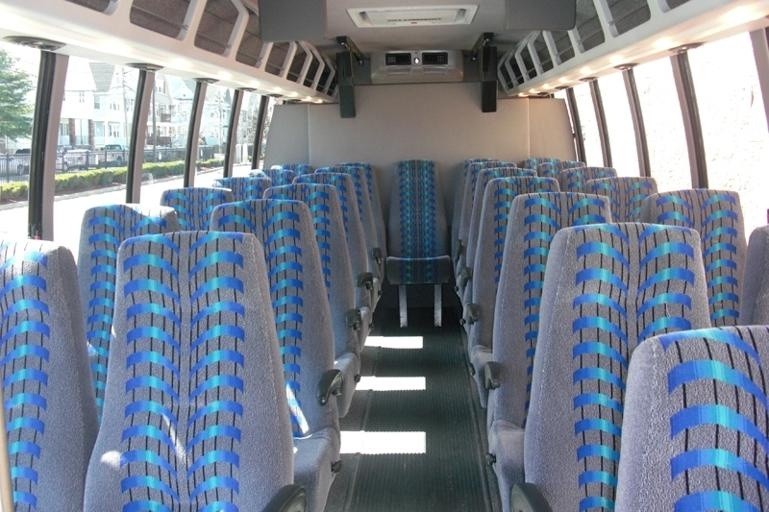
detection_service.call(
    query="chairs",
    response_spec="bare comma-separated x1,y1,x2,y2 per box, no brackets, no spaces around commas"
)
0,162,387,512
386,159,450,328
452,158,769,512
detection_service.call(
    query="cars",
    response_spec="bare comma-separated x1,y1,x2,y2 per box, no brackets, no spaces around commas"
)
0,153,24,176
13,141,220,176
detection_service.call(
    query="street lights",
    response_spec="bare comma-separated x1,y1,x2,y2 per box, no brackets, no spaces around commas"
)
215,92,222,153
